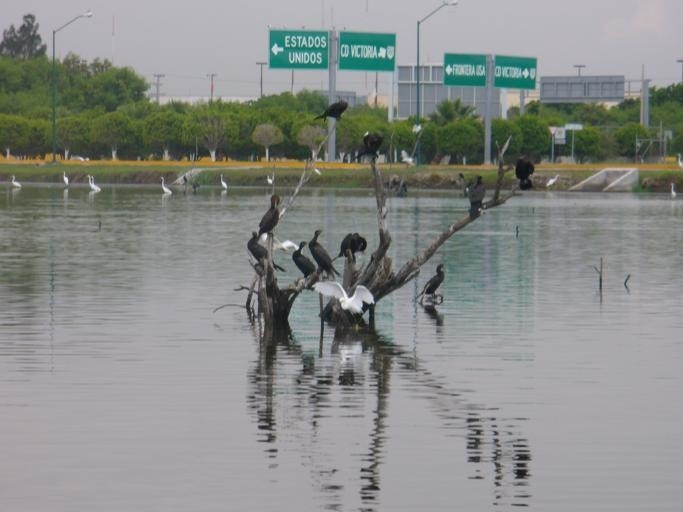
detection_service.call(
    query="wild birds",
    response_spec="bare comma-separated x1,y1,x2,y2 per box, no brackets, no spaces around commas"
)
400,150,416,167
63,170,69,187
546,174,560,188
10,174,20,188
248,229,368,280
257,194,281,237
676,152,682,168
16,180,19,181
419,263,445,305
86,173,101,193
468,175,486,218
219,173,228,189
516,153,535,189
159,177,172,195
669,182,676,196
363,132,384,159
310,281,376,315
313,101,349,122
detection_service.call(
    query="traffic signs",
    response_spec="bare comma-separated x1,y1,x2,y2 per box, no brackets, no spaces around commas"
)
443,52,486,88
493,54,536,91
268,29,329,71
336,30,396,72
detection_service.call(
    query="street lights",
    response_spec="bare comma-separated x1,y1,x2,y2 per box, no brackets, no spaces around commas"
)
415,1,460,166
153,74,164,104
50,11,96,159
257,62,268,99
207,73,218,102
572,64,587,77
